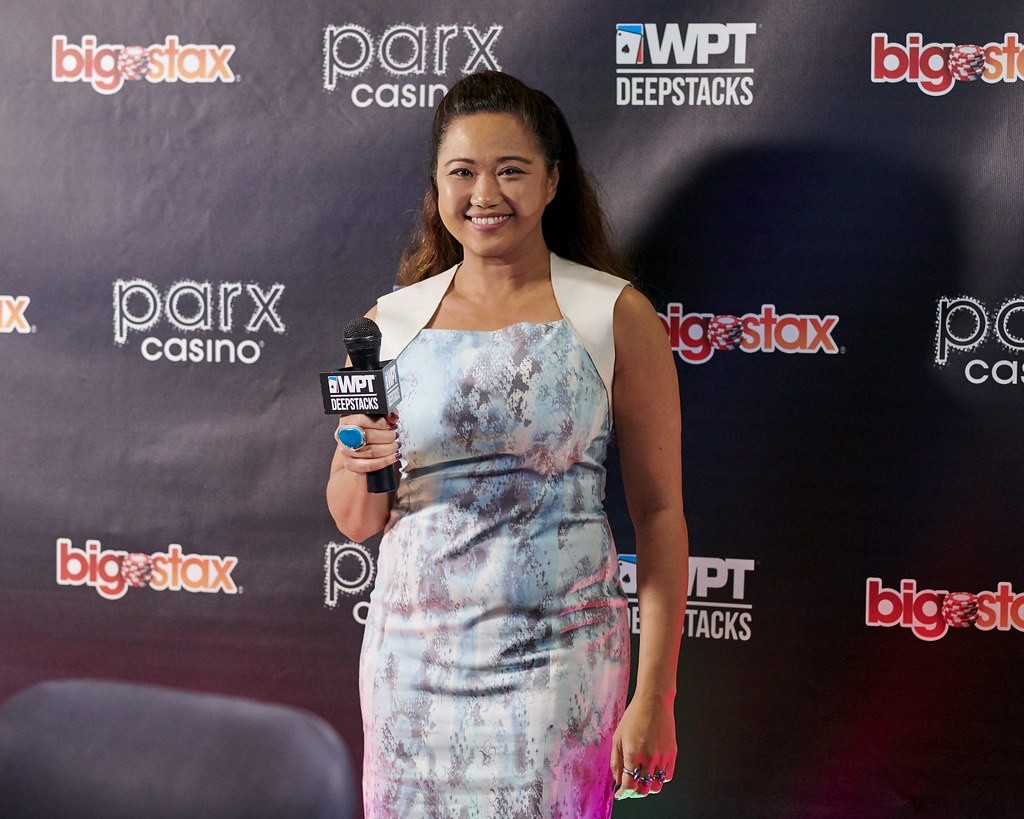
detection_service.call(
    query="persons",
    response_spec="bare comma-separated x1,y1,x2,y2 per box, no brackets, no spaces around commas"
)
327,72,690,819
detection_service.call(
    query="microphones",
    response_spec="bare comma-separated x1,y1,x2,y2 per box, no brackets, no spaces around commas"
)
320,317,403,493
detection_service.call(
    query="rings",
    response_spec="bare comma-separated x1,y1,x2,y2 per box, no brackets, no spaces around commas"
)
640,774,653,785
337,425,367,451
655,771,666,783
623,768,640,780
